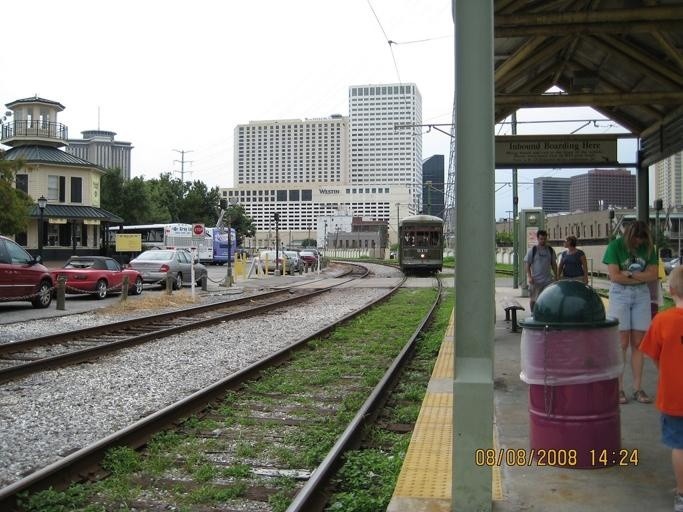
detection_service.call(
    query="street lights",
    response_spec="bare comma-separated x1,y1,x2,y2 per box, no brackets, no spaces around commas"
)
37,195,47,265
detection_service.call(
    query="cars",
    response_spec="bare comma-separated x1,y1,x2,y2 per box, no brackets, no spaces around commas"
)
253,239,321,275
663,257,681,275
127,249,207,291
0,235,54,308
50,256,144,300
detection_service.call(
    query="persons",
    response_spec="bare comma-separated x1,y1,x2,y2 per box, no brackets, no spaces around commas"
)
524,230,557,311
557,236,589,285
601,220,659,404
638,264,683,511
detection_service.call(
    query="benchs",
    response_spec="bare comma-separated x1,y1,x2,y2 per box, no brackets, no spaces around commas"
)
500,299,525,332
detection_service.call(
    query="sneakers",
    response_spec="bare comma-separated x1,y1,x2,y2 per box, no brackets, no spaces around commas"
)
618,390,627,404
672,493,683,511
631,390,652,403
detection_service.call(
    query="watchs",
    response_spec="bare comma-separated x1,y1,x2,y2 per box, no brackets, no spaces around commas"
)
628,270,633,278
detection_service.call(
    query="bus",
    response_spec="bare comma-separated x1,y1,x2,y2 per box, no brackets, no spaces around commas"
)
104,223,192,262
200,227,236,265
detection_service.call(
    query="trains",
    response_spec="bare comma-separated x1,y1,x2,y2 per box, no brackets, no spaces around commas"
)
398,214,443,273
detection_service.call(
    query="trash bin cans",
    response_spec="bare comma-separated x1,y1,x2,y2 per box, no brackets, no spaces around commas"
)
518,280,623,470
